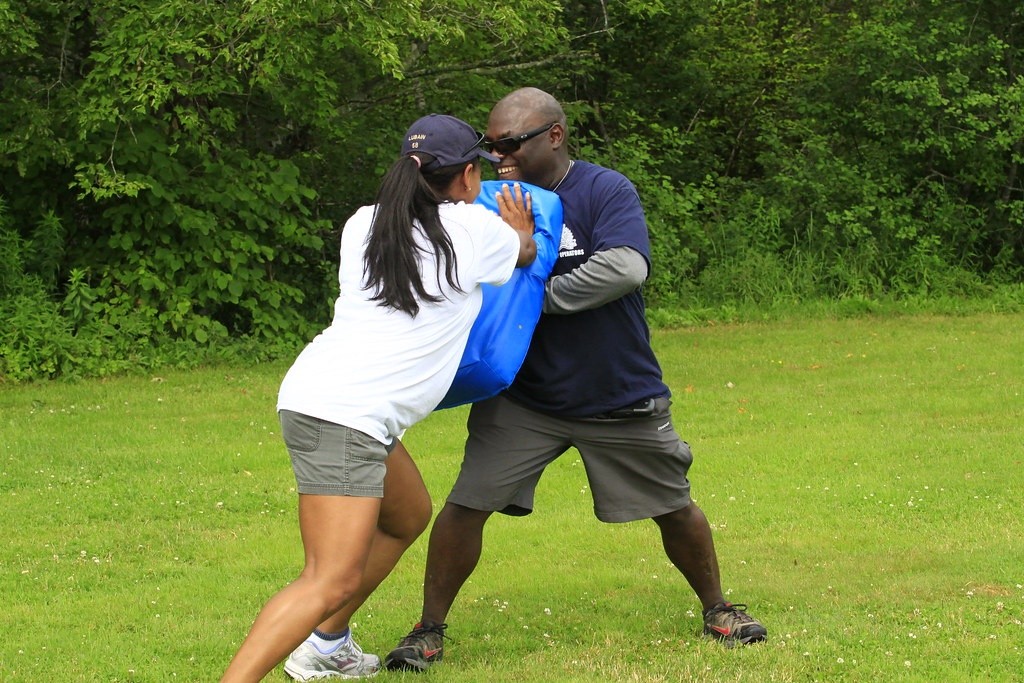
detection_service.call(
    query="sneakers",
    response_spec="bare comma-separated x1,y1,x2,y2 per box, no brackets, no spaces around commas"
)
385,619,452,670
701,602,768,648
284,625,382,681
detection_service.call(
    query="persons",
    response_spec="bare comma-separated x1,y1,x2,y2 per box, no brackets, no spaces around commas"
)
383,87,766,671
213,111,540,683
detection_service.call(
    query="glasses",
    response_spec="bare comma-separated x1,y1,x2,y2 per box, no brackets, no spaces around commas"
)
484,121,556,155
462,131,484,157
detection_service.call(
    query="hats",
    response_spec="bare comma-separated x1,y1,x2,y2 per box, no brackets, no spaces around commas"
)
400,112,500,172
